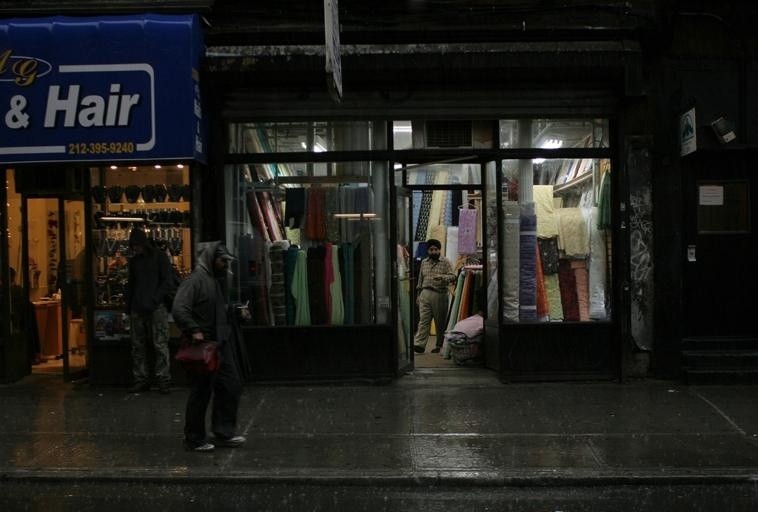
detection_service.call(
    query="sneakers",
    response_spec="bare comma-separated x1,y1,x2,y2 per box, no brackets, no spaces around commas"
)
183,439,215,453
209,433,247,448
128,383,170,394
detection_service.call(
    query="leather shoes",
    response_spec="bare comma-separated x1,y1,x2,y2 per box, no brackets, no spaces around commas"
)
413,345,440,354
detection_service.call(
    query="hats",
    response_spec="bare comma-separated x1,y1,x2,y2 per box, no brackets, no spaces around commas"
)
213,247,234,260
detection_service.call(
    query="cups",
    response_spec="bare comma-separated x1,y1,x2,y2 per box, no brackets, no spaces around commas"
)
236,305,249,323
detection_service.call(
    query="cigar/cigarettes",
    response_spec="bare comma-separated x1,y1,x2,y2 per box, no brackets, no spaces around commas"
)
225,269,234,276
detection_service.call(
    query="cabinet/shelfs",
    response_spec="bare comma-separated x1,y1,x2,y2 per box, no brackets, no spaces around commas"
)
31,299,71,361
91,200,192,279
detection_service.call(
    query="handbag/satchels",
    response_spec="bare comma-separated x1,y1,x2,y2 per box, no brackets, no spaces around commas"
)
175,340,220,374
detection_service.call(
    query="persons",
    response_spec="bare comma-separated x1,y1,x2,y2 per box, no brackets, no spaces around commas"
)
123,229,176,395
173,242,250,451
10,267,39,378
414,239,456,354
